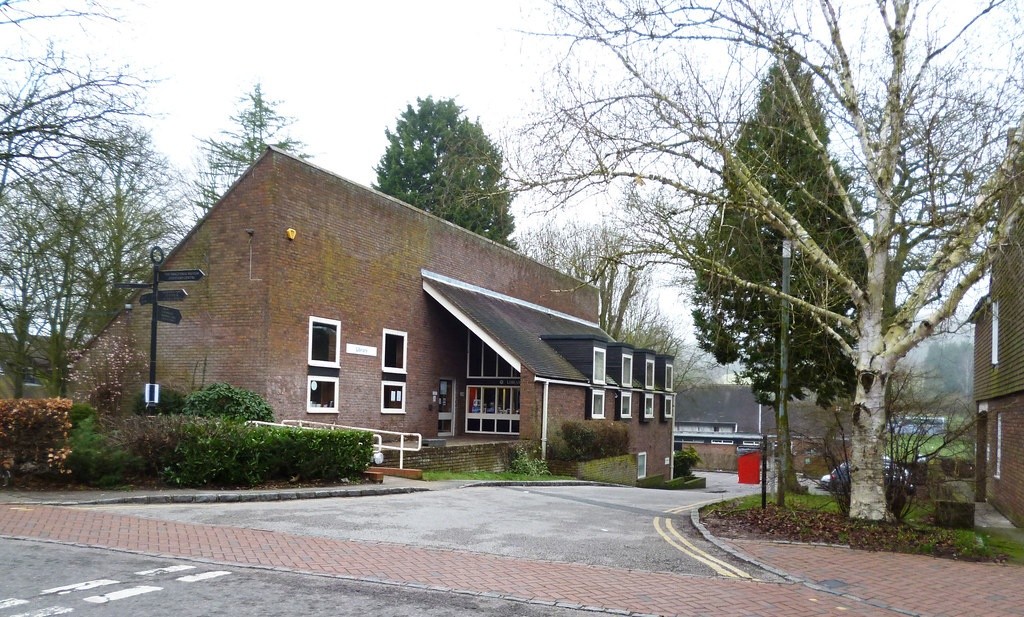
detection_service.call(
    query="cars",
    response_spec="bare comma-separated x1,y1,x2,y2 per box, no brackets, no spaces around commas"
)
820,458,916,498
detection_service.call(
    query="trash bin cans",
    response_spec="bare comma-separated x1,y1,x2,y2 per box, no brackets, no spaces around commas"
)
736,449,761,484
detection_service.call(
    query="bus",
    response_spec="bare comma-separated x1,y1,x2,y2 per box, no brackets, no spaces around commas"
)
887,415,948,438
